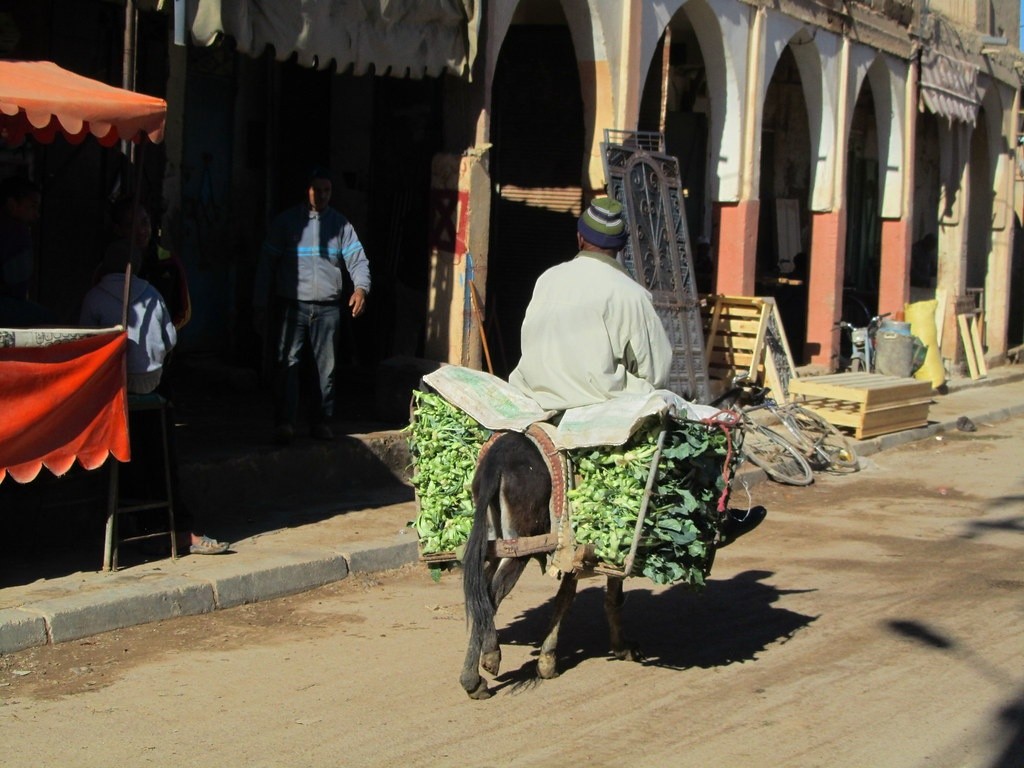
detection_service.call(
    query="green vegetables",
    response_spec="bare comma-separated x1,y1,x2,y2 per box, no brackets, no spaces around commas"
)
408,388,727,586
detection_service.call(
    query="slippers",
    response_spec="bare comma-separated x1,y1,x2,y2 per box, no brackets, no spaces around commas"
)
184,535,230,554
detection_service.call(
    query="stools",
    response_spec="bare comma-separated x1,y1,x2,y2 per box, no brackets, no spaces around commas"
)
110,393,178,572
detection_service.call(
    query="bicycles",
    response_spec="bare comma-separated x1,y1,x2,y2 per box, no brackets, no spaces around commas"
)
711,373,860,484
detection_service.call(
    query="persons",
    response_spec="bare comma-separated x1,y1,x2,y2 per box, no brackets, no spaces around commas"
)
80,240,229,559
0,174,62,328
253,174,371,445
910,232,936,286
110,197,192,330
509,198,767,536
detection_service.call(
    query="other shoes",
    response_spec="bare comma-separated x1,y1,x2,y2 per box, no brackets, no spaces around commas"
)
713,507,768,550
275,422,296,443
309,422,335,441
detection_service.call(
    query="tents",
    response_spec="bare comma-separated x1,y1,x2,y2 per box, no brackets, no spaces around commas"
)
0,60,167,148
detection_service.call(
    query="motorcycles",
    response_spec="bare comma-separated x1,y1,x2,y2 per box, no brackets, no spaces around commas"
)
832,313,893,377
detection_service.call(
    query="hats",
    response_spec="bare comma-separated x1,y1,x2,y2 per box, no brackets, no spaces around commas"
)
578,195,632,250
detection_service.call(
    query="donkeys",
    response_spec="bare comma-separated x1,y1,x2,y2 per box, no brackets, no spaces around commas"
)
460,383,773,700
